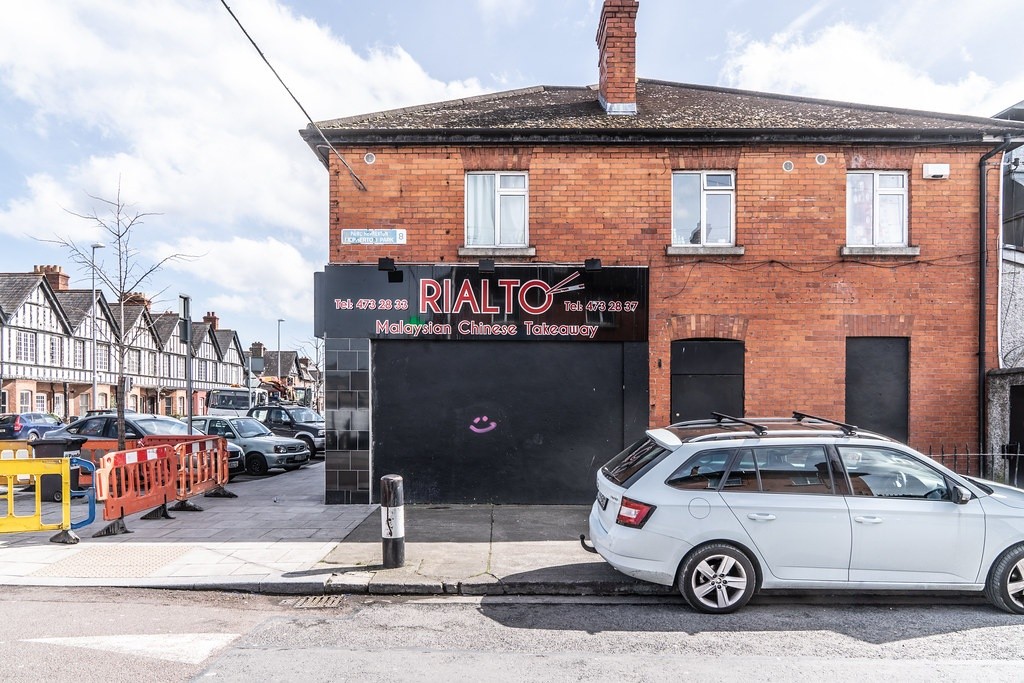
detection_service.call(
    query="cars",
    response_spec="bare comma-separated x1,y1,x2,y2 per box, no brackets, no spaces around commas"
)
85,410,138,417
581,409,1024,617
2,413,66,441
181,416,311,475
40,413,246,482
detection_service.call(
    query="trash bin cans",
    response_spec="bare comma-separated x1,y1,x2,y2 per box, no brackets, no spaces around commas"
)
26,435,88,502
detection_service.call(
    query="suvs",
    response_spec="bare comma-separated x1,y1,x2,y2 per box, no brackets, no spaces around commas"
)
246,403,326,459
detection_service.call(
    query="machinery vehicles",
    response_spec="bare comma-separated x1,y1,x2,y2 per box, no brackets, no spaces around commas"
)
206,384,269,417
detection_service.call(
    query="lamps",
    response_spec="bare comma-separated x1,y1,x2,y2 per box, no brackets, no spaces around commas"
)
585,258,602,272
478,259,495,273
378,258,394,270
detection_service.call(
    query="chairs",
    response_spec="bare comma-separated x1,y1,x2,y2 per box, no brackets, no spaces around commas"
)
224,425,232,433
273,411,283,424
208,426,218,435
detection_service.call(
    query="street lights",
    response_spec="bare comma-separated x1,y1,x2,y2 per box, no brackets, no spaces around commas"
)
91,242,106,416
278,318,285,397
